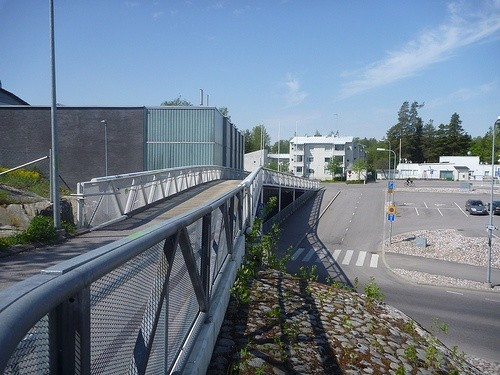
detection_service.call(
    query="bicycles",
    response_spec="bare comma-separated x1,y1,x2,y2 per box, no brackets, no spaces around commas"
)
403,180,415,187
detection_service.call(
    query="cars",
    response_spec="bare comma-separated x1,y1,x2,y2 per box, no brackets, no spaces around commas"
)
486,201,500,216
465,199,487,216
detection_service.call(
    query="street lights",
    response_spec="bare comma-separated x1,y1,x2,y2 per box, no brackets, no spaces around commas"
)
100,119,108,178
487,115,500,280
376,147,397,245
